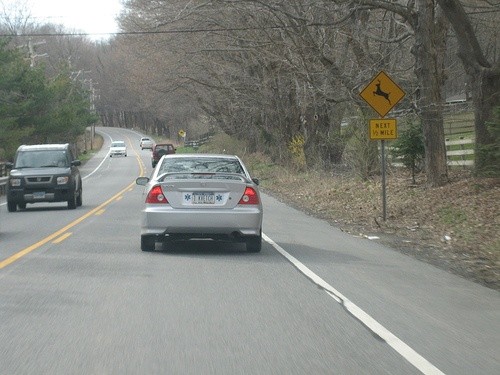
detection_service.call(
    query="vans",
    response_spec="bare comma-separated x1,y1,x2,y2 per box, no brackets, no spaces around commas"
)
5,144,82,210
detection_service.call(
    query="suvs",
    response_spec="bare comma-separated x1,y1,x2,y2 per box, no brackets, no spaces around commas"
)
150,143,177,167
139,136,150,146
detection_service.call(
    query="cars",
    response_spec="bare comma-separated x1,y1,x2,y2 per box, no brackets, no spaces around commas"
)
135,154,265,253
108,141,127,158
141,142,155,149
